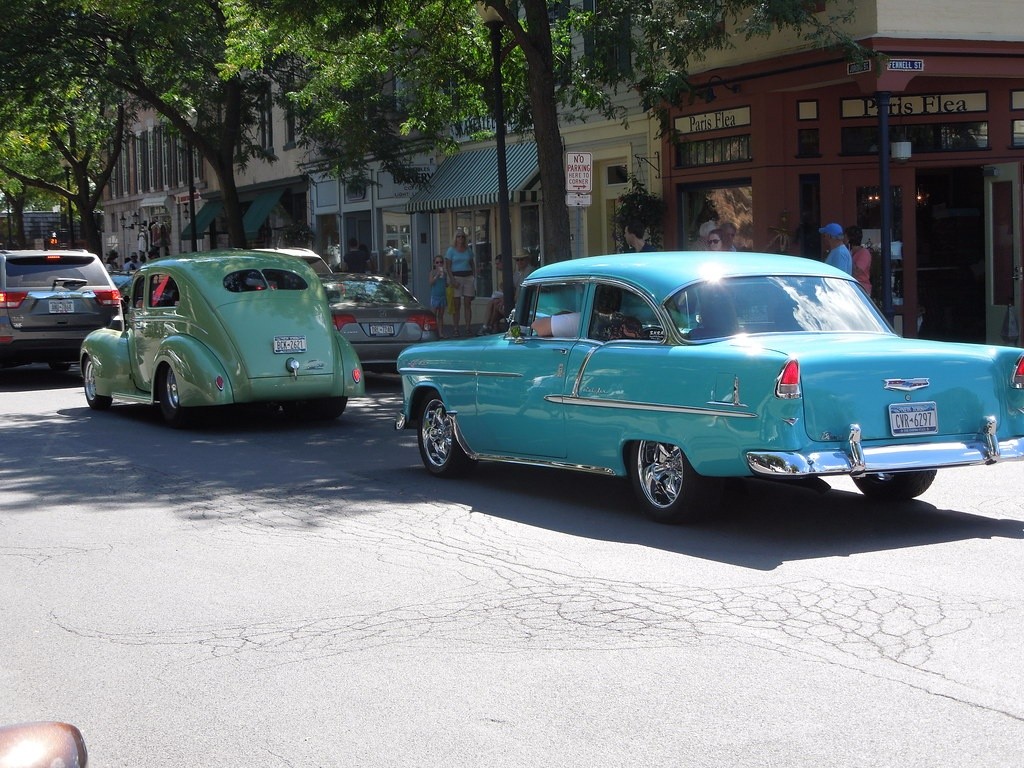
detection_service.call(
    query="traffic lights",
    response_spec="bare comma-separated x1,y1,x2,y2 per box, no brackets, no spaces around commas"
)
48,231,66,246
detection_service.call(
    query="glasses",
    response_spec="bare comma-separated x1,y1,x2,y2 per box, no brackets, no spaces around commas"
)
516,258,524,261
457,235,465,238
436,261,443,264
131,256,136,258
707,239,721,244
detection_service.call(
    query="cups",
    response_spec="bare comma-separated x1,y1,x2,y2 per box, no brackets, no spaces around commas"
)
438,267,444,276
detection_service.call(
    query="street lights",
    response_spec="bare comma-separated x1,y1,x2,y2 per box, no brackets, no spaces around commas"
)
60,158,76,248
474,1,515,315
185,107,198,252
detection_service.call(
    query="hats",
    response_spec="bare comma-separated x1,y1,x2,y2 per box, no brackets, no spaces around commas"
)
511,249,530,258
819,223,843,237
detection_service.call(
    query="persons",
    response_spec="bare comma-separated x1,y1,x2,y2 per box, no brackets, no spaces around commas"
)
428,255,450,339
476,249,539,335
689,221,738,252
844,225,872,301
341,237,377,275
100,249,163,271
819,223,852,277
444,230,476,338
673,285,750,342
623,219,660,253
532,285,642,342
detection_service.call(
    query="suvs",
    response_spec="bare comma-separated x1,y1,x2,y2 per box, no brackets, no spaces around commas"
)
249,248,335,274
0,248,124,373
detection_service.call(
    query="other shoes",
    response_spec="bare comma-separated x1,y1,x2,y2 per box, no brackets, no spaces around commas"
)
490,327,501,334
477,328,489,335
452,330,460,337
440,335,448,339
464,329,472,336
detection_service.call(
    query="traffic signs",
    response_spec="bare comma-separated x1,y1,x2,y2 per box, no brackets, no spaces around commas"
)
567,152,592,191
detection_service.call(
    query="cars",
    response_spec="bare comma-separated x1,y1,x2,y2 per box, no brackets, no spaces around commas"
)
80,248,364,428
316,273,440,373
397,251,1024,526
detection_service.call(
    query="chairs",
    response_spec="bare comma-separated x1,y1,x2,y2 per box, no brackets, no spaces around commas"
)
597,316,642,339
688,302,740,338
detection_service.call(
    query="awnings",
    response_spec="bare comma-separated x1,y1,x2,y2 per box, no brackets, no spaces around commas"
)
177,190,288,241
405,137,566,215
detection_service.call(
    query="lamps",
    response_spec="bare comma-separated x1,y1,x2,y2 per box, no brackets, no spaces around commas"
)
642,85,679,114
891,125,911,159
183,203,191,220
132,212,147,226
119,215,134,229
685,75,740,106
